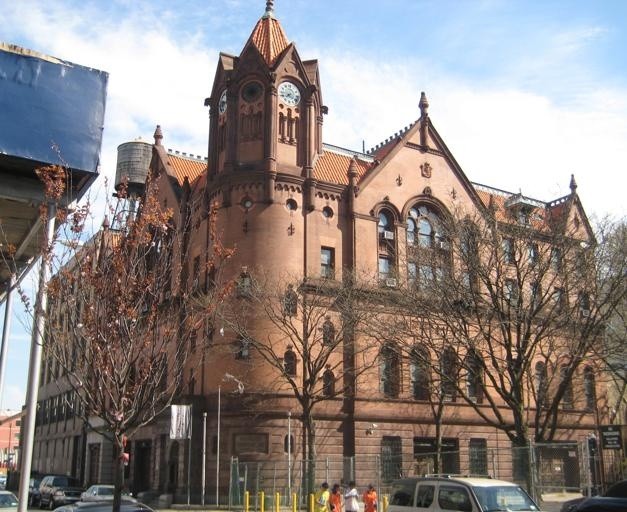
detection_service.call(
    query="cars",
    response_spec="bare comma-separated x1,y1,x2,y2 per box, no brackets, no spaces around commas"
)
574,496,626,512
559,477,626,512
0,474,154,512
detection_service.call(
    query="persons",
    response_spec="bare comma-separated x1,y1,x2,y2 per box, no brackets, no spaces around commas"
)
343,481,359,512
330,483,343,512
361,483,378,512
314,482,331,512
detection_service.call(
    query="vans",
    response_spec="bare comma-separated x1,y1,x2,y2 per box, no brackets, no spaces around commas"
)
385,473,542,511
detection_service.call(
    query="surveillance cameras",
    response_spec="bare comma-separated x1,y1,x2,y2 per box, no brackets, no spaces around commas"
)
371,423,378,430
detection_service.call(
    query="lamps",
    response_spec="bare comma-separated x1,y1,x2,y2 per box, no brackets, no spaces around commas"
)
366,423,379,437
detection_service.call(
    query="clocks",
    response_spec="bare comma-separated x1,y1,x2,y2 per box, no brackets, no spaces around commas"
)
217,89,228,114
278,81,301,107
